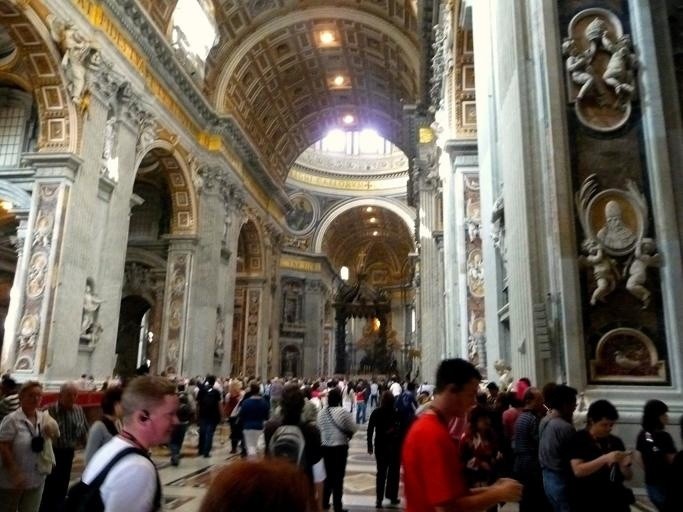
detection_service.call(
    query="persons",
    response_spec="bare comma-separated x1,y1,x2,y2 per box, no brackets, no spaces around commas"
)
82,286,107,334
602,29,638,95
0,358,683,512
595,200,634,250
561,38,603,100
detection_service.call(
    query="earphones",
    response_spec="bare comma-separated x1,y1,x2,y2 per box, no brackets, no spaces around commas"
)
142,410,149,422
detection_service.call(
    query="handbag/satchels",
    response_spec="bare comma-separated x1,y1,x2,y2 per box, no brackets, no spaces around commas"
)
38,411,56,475
177,402,193,422
589,464,636,512
343,428,353,440
62,447,161,512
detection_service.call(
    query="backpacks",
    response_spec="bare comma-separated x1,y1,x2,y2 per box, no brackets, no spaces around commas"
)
266,419,315,471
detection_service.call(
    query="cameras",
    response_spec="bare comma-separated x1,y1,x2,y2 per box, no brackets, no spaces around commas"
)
624,449,632,456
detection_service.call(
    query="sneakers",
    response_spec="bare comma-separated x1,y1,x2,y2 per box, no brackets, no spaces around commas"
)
170,449,247,466
349,403,379,425
323,495,401,512
